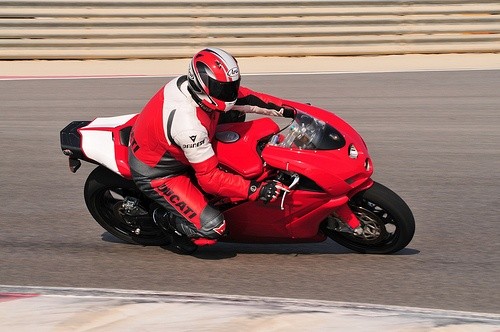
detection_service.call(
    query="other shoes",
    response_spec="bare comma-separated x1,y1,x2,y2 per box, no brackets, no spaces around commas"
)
152,208,197,254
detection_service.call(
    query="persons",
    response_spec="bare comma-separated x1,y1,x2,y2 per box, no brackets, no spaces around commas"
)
128,49,312,254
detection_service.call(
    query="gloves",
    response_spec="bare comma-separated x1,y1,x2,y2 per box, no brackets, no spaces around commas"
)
248,181,293,204
293,101,312,128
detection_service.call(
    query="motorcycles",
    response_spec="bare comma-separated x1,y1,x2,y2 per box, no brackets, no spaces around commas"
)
60,102,415,255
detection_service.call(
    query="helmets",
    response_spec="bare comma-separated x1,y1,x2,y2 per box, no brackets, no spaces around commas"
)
187,47,242,114
